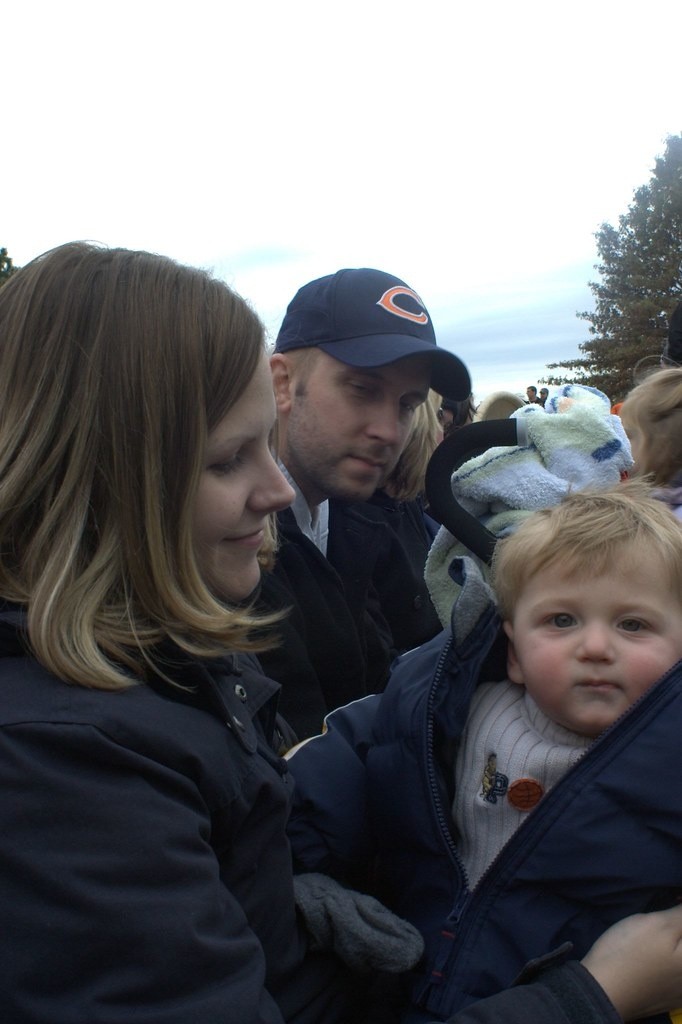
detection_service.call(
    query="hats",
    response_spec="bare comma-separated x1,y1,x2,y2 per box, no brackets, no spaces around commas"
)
273,268,471,401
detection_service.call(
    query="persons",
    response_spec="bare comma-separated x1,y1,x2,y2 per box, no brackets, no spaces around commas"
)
235,269,440,742
378,268,682,628
0,243,682,1024
280,480,682,1024
